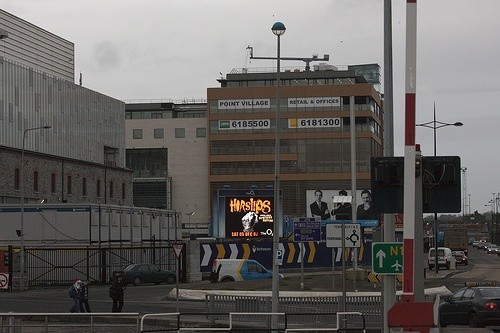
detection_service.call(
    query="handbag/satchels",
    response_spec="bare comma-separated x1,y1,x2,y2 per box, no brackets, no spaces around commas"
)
109,281,117,299
69,285,75,298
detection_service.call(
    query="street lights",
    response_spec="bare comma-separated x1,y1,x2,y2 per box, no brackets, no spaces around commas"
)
484,192,500,215
467,193,471,217
18,126,53,292
271,22,286,333
415,101,464,275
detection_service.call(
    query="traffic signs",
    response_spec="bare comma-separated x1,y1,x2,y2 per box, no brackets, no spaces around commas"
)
371,242,405,274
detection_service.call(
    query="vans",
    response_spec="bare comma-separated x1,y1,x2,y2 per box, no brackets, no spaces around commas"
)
208,258,284,290
427,248,455,270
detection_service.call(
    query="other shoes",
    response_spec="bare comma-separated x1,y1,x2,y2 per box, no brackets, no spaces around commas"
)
69,310,73,313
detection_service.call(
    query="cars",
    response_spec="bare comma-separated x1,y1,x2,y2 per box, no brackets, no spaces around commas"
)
467,238,500,256
451,250,468,266
438,281,500,333
112,263,175,287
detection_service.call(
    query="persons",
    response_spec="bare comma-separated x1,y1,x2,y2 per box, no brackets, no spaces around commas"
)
70,278,92,313
112,274,125,313
310,190,330,220
331,190,352,220
357,190,373,219
